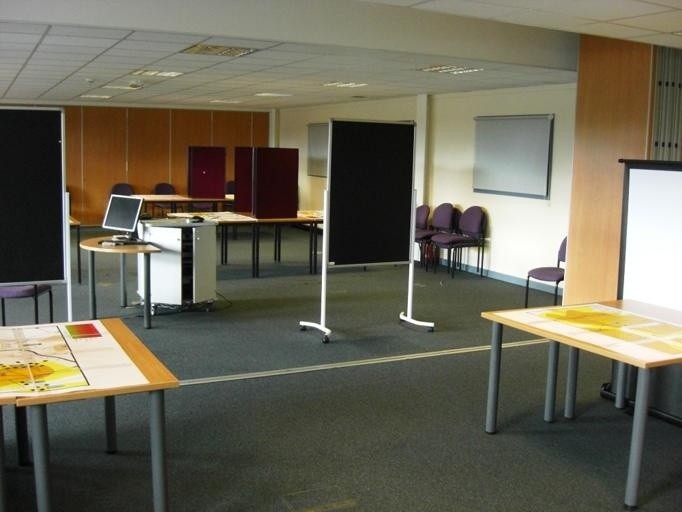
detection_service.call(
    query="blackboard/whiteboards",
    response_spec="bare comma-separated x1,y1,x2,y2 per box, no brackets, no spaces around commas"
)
0,106,66,287
322,118,416,268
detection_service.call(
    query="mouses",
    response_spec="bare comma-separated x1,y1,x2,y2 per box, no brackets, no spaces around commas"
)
136,239,145,244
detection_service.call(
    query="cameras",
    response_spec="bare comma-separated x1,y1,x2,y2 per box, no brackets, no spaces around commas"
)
101,193,144,239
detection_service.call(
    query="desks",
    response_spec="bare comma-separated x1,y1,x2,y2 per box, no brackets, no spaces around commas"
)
480,297,682,510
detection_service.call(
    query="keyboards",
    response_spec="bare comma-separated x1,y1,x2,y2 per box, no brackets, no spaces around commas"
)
98,240,129,245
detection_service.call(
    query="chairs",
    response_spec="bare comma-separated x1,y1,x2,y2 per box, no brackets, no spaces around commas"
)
415,203,487,279
152,183,184,218
225,181,236,210
109,183,135,195
525,236,566,308
